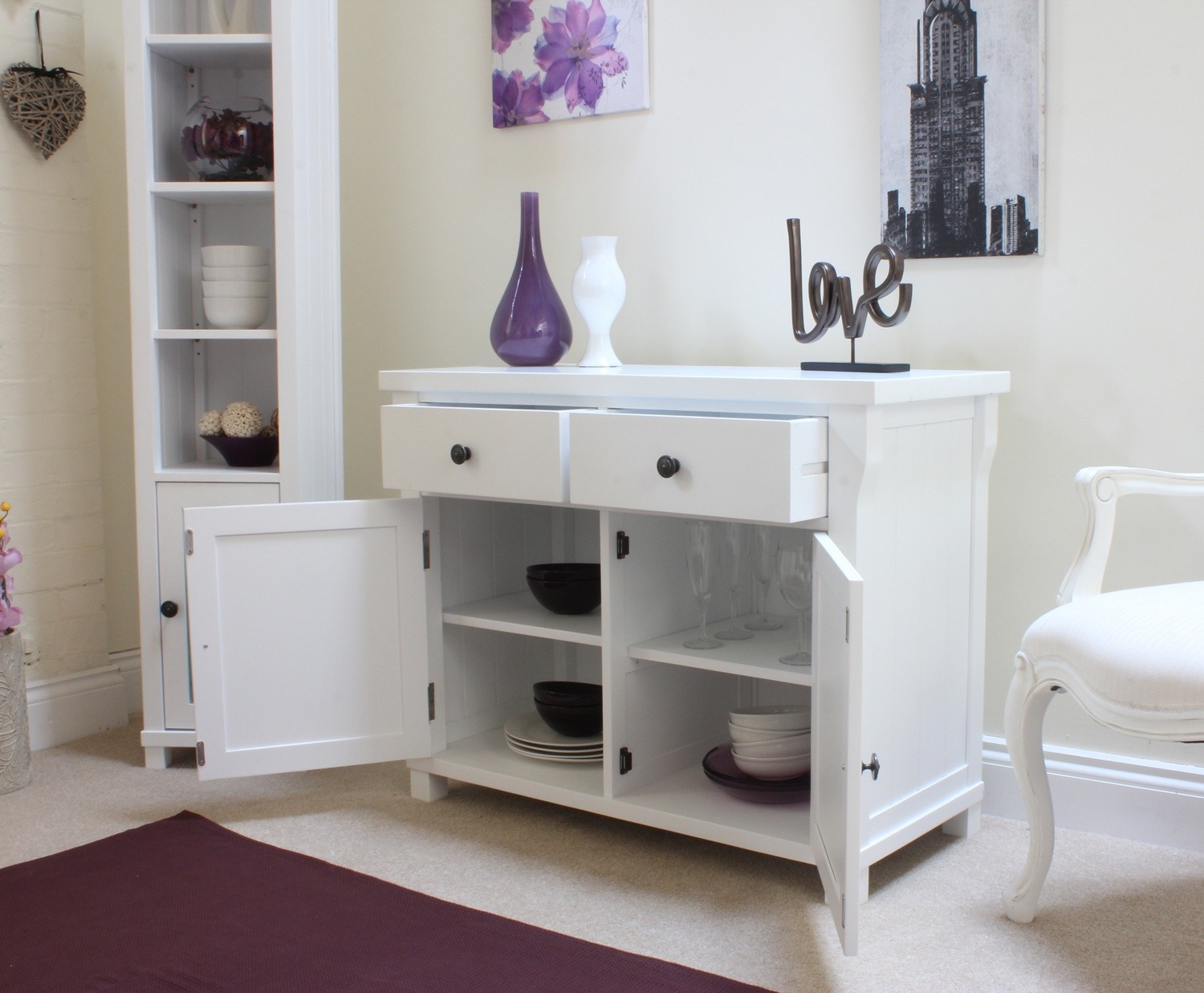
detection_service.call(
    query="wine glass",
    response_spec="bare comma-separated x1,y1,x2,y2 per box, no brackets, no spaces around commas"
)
681,522,812,666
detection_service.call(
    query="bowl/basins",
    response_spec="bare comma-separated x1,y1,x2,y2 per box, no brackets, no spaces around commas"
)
199,434,278,468
200,244,271,329
526,562,600,614
700,703,810,804
532,680,602,736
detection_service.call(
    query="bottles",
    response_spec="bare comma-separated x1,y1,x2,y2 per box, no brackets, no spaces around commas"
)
489,193,572,367
175,94,274,180
572,234,625,368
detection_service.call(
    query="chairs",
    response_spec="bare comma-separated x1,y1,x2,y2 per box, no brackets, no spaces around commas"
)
1002,466,1204,923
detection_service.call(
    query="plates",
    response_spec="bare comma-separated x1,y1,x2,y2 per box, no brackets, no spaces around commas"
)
504,713,603,764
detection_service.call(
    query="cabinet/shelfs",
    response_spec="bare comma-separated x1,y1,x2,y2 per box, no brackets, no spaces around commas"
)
183,364,1010,952
120,0,343,768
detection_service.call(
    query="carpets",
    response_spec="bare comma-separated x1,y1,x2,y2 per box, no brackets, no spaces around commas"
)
1,811,777,992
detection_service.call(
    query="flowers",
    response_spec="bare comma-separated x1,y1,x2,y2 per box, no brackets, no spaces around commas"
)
0,502,24,632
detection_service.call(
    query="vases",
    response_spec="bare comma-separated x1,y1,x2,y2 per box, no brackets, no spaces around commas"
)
489,192,574,367
572,234,626,368
0,633,30,795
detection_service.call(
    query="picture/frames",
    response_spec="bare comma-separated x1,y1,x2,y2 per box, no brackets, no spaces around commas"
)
879,1,1046,261
